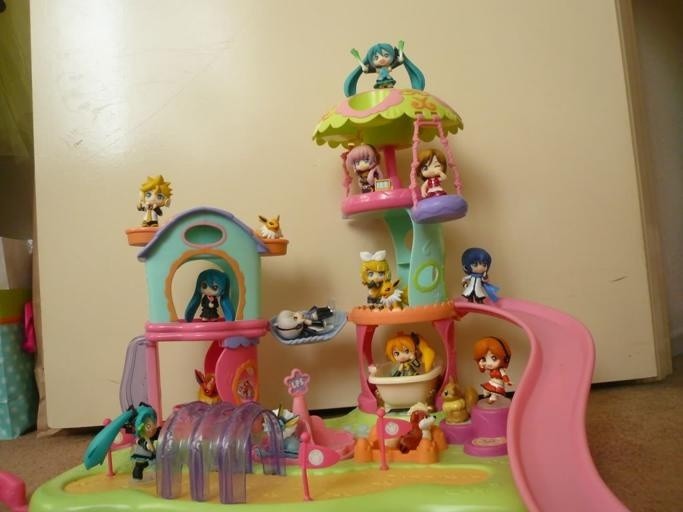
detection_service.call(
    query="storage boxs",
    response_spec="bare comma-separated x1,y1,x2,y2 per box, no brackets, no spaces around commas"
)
0,236,39,442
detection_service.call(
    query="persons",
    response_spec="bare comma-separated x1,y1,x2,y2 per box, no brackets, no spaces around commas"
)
0,37,631,512
84,404,161,479
137,175,334,340
344,41,512,404
